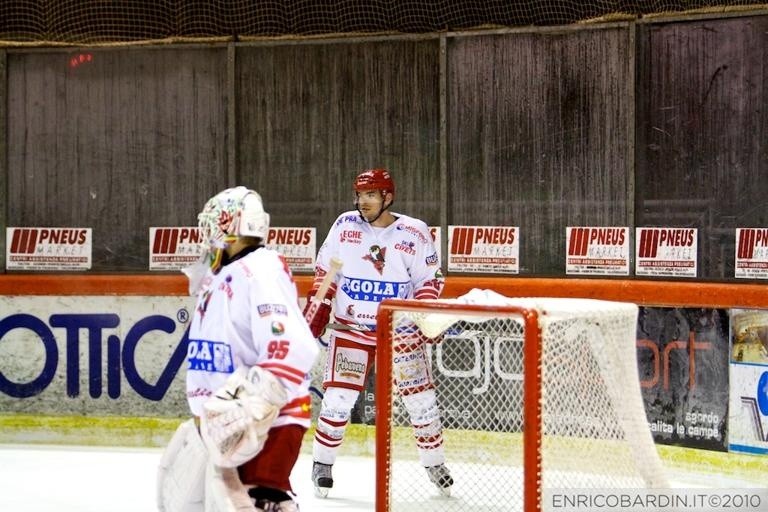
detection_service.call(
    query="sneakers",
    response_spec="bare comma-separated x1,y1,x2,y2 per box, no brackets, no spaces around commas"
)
310,461,333,488
426,463,454,489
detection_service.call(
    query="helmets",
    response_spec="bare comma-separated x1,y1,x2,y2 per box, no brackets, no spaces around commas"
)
196,184,270,275
353,170,394,210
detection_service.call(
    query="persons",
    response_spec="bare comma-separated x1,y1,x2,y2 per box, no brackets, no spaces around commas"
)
156,183,322,512
303,169,453,489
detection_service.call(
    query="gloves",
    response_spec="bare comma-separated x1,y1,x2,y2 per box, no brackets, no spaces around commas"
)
303,289,332,338
